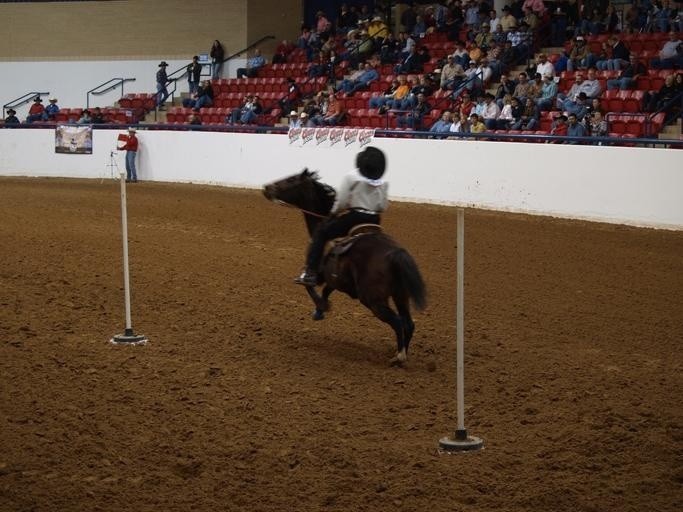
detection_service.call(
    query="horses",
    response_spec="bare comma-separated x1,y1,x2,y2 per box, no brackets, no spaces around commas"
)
262,166,428,365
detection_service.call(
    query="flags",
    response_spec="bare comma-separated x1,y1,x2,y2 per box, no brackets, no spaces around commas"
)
117,133,127,141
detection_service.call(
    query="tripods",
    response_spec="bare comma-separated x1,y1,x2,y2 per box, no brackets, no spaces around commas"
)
101,152,121,184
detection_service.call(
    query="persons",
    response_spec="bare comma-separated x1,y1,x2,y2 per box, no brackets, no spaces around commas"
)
26,96,44,122
156,61,169,111
231,95,262,124
91,107,103,123
76,109,91,123
183,81,214,110
210,40,224,79
237,48,265,78
117,126,138,183
187,56,201,93
1,108,19,129
41,109,49,121
45,97,58,112
274,0,682,145
294,146,388,286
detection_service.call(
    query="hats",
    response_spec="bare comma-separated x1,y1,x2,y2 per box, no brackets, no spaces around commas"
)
462,91,496,100
481,21,531,28
158,61,169,67
500,5,513,12
126,127,138,133
576,91,591,100
283,76,297,83
448,54,490,66
356,147,386,180
6,97,57,115
356,16,383,24
573,35,588,44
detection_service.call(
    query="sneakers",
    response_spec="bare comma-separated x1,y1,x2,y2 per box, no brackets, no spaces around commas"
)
126,179,137,183
293,271,320,287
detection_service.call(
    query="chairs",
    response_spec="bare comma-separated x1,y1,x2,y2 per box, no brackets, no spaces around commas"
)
56,31,683,150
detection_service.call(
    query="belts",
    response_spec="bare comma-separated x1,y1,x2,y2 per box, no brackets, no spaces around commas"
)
353,207,382,215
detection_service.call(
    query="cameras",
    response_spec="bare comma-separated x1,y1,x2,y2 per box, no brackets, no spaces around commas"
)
584,105,595,120
